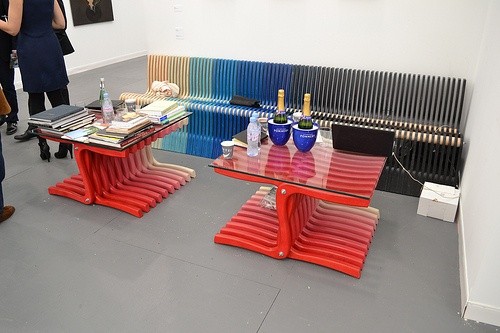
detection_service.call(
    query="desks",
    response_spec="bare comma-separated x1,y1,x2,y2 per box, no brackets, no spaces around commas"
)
207,119,388,279
29,105,195,217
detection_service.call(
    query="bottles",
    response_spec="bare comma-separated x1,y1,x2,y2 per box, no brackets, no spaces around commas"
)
274,89,287,124
252,111,261,151
247,117,259,156
101,93,115,123
99,78,105,104
298,93,313,129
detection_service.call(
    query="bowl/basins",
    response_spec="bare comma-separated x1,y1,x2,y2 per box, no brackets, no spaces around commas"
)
291,121,319,152
266,117,294,146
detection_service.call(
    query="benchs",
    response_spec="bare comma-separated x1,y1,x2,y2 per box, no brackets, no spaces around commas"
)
119,54,466,199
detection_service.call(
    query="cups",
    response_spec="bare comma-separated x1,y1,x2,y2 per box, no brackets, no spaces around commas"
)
115,108,128,116
133,105,141,112
257,118,268,134
221,140,234,159
125,99,136,112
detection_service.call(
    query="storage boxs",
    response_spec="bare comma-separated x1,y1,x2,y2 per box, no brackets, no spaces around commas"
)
416,180,461,222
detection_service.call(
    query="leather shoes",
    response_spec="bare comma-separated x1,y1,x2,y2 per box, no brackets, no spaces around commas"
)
14,126,39,141
6,122,17,135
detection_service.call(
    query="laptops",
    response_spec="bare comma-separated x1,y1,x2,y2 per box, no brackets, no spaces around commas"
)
331,124,396,156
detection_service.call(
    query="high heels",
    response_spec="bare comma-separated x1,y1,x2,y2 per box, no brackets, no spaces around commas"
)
38,141,51,162
54,142,73,159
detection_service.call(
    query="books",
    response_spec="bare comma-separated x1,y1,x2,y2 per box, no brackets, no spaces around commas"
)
85,98,123,110
28,104,111,144
138,100,186,123
88,111,155,151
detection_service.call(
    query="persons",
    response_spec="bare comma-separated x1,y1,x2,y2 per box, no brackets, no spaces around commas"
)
0,82,15,223
0,0,74,161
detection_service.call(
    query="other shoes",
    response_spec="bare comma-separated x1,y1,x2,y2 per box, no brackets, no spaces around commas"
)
0,206,15,223
